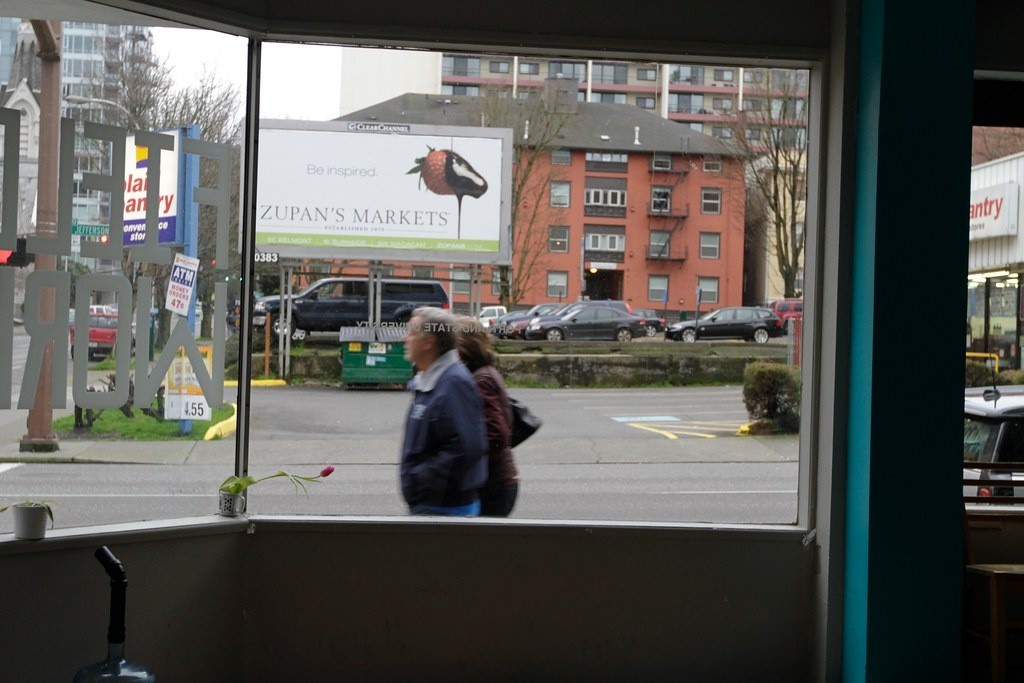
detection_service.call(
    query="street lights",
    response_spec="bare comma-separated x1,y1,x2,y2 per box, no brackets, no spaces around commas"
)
65,95,141,130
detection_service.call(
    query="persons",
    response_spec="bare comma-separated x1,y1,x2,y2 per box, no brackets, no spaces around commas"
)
458,317,520,517
400,306,487,516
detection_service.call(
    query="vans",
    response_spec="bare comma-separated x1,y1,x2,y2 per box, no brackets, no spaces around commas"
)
253,278,449,342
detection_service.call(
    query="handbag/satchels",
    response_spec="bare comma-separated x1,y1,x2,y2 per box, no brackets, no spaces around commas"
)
509,397,542,450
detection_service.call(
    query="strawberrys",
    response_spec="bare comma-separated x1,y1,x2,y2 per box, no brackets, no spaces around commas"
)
406,145,487,197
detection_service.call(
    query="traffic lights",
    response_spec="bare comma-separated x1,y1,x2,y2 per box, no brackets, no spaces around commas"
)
100,235,108,244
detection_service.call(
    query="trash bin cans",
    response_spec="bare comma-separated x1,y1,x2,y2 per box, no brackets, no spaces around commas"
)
339,327,415,388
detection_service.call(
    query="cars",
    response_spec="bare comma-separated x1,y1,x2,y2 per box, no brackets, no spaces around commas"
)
473,306,508,328
769,300,803,335
526,300,636,340
489,304,567,340
633,309,666,338
530,307,645,343
504,309,560,340
964,391,1024,506
665,307,783,344
69,313,119,359
